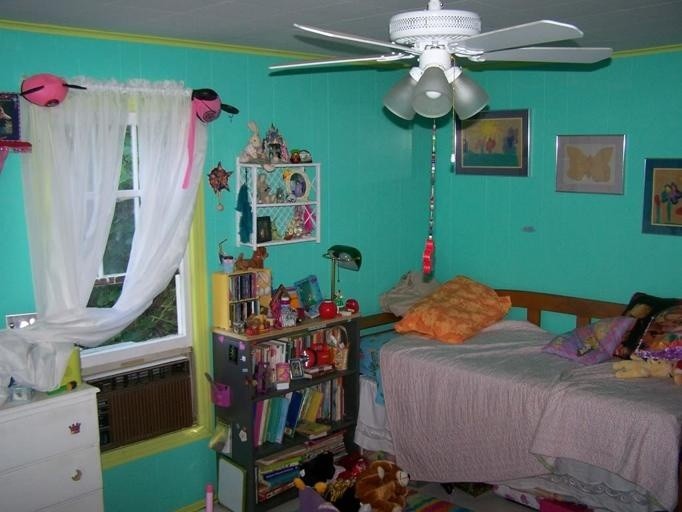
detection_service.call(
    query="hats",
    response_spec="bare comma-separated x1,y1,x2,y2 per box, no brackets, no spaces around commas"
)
20,74,86,107
192,89,238,123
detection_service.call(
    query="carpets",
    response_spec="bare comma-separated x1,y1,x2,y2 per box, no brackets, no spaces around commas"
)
400,485,475,512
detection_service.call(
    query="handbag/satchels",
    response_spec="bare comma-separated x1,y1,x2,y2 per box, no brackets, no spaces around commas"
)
299,450,335,487
326,326,350,371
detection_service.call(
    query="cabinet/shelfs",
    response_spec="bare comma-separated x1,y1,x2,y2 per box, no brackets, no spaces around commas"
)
212,272,259,326
212,318,361,511
0,377,104,512
235,157,321,251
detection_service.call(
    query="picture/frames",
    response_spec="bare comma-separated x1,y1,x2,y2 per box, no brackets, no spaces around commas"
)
454,109,530,177
641,159,682,235
287,357,305,381
0,93,20,140
294,273,325,316
554,134,625,195
284,165,311,202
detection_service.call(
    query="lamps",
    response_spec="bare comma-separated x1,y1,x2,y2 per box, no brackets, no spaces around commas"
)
323,246,362,303
384,52,489,122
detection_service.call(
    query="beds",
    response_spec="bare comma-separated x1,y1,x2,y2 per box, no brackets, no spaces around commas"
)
352,290,682,511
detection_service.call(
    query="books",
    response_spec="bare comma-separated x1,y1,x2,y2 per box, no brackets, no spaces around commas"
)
296,274,325,318
230,274,260,324
252,326,349,503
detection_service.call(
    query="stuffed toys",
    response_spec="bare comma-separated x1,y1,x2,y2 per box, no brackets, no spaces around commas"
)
232,244,269,274
270,284,298,329
257,172,276,203
292,450,410,511
240,121,263,164
613,353,681,384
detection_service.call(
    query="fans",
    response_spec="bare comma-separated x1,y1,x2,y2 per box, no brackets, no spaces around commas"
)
269,0,613,121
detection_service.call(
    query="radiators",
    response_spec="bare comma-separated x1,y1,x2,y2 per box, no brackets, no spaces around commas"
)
85,356,194,452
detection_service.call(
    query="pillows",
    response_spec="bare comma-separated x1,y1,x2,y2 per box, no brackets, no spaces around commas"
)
545,291,680,365
395,276,511,345
